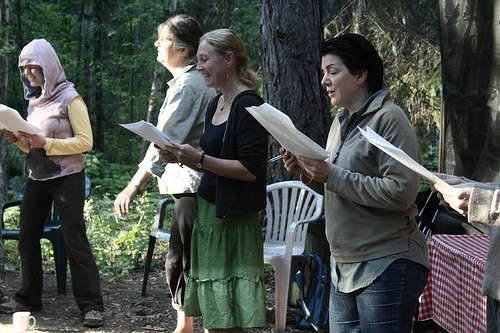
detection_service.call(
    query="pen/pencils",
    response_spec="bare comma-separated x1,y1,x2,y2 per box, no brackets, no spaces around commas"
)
269,154,290,164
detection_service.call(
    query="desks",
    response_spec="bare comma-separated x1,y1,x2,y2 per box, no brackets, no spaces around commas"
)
416,233,491,333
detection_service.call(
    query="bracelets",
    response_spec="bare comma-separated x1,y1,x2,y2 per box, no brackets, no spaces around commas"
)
128,181,141,190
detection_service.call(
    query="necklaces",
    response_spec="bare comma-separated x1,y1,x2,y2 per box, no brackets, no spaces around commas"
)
219,85,245,112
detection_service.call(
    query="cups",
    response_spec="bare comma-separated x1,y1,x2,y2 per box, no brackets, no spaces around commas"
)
13,311,37,332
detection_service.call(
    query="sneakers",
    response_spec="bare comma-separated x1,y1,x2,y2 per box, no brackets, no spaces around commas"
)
0,300,34,314
84,310,104,326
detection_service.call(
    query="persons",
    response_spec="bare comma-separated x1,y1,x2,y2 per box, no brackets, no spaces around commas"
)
431,173,500,333
114,15,218,333
154,28,269,333
0,39,104,327
280,33,430,333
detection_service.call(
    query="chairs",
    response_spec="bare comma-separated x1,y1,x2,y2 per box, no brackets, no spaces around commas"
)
0,175,92,298
139,181,323,333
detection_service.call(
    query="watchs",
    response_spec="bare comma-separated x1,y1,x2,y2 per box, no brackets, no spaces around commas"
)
196,153,206,168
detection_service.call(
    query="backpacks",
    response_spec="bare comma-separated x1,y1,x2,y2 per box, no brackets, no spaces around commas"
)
294,252,330,331
